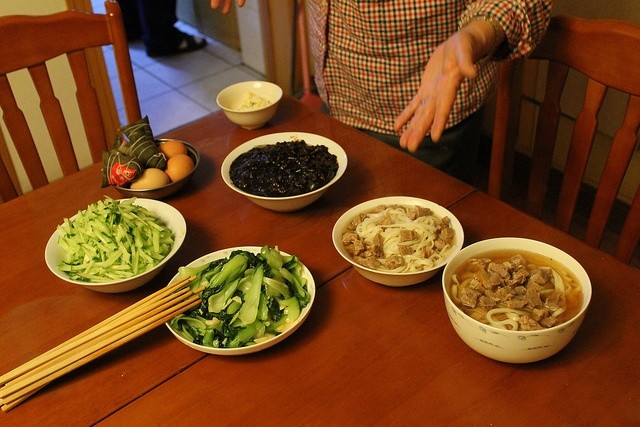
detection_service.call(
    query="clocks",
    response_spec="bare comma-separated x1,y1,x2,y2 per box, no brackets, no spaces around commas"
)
168,245,309,348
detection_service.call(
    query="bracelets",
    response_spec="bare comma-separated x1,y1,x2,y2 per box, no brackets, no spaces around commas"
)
440,235,593,366
46,196,191,293
213,79,284,129
113,133,199,194
220,131,349,209
330,195,464,286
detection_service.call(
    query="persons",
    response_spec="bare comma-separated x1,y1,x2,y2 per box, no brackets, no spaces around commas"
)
210,0,555,188
115,0,207,58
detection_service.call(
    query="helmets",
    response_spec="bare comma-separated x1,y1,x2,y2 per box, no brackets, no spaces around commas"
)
164,242,319,354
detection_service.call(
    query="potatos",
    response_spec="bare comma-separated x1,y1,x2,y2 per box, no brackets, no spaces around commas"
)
57,193,171,282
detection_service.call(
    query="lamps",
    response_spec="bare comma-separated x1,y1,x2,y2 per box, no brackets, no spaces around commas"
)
1,1,142,207
487,13,640,264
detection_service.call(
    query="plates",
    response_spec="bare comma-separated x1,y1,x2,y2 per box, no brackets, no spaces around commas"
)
174,0,240,51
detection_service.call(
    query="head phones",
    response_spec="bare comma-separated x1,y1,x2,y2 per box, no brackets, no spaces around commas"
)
0,271,207,413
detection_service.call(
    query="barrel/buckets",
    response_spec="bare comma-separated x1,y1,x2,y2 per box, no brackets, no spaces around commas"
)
146,30,208,58
